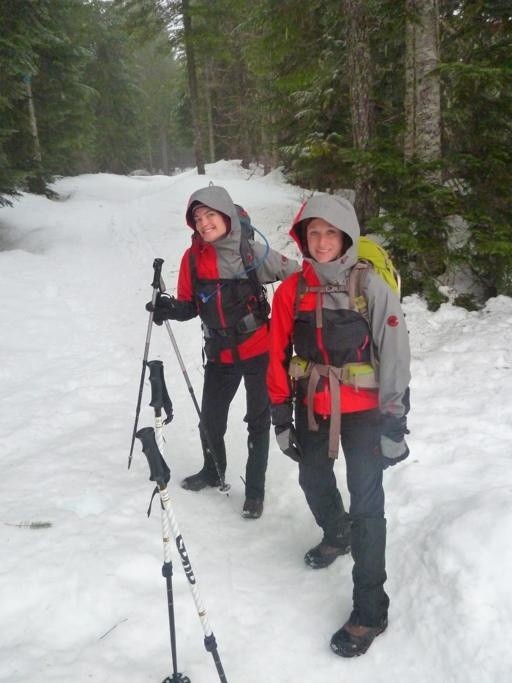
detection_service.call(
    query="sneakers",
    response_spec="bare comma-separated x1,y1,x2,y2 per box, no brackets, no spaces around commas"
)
330,618,387,658
182,474,225,491
304,542,352,569
242,498,263,518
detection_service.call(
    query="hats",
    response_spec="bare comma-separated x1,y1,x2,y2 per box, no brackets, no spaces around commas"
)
190,201,207,216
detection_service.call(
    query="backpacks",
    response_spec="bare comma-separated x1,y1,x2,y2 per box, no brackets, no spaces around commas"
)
287,236,401,390
189,205,272,338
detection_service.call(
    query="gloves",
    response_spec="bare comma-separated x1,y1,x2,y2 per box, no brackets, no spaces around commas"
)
374,414,409,470
269,402,303,462
146,296,196,325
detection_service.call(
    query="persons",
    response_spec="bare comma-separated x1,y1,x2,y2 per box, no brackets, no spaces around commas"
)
266,192,412,657
146,185,300,524
